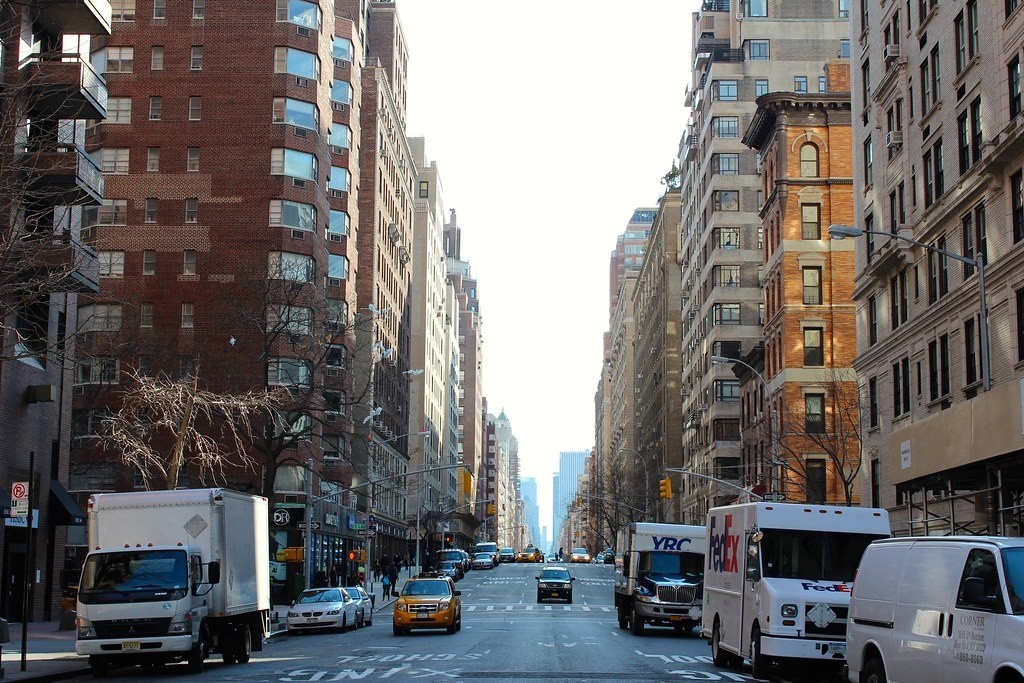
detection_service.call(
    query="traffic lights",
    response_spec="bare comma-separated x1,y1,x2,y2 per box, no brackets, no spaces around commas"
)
350,550,365,563
574,530,579,537
659,480,671,499
444,534,454,542
488,504,496,514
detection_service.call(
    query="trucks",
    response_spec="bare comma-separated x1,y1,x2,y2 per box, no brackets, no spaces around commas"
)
701,502,892,677
615,525,707,634
60,486,268,676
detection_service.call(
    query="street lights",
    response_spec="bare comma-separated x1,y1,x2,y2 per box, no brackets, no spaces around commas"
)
712,356,778,502
441,477,488,550
828,225,997,541
365,430,430,591
618,448,648,523
416,453,463,574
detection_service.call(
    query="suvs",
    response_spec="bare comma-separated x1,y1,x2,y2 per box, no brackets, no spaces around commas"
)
390,573,462,636
536,566,576,602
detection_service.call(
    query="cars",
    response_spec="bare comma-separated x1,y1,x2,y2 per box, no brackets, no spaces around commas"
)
516,544,546,563
591,548,614,564
569,547,590,562
429,542,516,582
285,584,372,632
547,553,564,562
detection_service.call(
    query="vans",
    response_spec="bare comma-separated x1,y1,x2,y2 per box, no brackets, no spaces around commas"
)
844,533,1023,683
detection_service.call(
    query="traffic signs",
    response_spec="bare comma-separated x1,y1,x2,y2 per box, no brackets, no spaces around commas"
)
297,522,322,531
